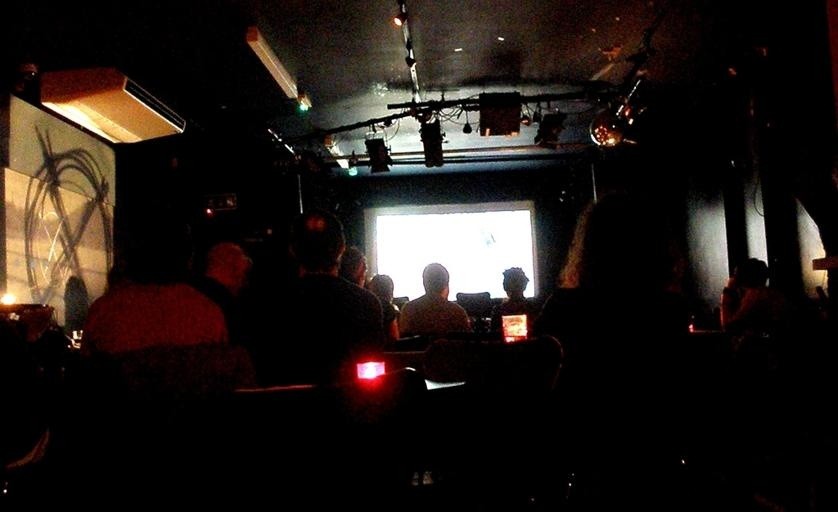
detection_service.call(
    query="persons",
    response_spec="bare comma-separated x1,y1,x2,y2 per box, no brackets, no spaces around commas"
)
79,190,789,390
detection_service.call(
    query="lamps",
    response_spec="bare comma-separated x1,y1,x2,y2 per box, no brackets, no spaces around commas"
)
534,113,569,149
589,104,628,149
365,138,392,174
419,119,443,169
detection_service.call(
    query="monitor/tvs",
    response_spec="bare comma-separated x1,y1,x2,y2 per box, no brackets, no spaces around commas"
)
501,314,529,344
